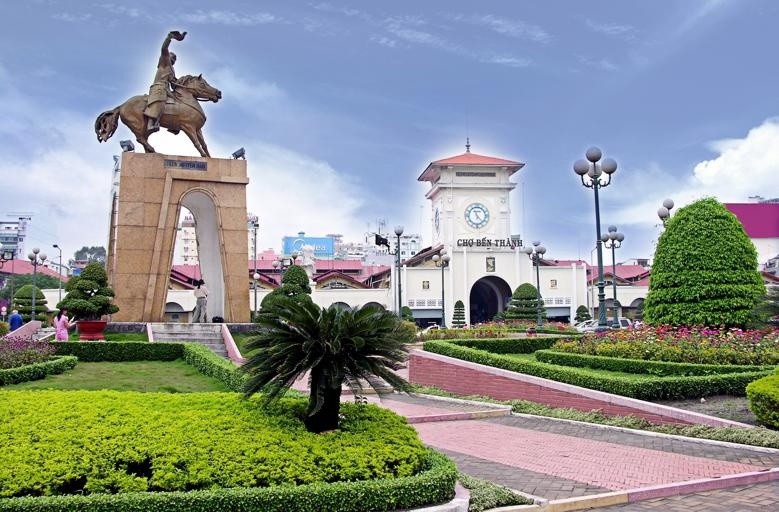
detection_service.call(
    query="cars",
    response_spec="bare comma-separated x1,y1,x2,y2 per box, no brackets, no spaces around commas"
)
573,316,633,334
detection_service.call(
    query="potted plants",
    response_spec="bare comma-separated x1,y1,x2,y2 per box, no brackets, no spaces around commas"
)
56,261,120,341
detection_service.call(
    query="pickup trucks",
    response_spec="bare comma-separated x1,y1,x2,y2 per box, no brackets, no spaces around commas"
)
413,324,440,335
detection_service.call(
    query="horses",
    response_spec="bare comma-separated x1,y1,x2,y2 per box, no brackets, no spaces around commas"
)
94,73,222,158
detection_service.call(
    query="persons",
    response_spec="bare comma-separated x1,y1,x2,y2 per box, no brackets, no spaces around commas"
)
143,32,177,131
54,307,71,342
8,309,23,332
191,278,208,323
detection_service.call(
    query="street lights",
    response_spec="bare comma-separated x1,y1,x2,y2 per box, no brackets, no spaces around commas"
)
523,239,546,327
375,223,405,320
253,270,260,322
432,249,451,329
292,251,298,266
573,145,625,331
271,255,292,288
28,247,47,320
53,244,62,306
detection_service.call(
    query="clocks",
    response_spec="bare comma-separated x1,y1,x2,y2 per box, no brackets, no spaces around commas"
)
465,202,490,229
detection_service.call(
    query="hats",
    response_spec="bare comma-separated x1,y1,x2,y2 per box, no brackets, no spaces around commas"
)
198,279,204,285
